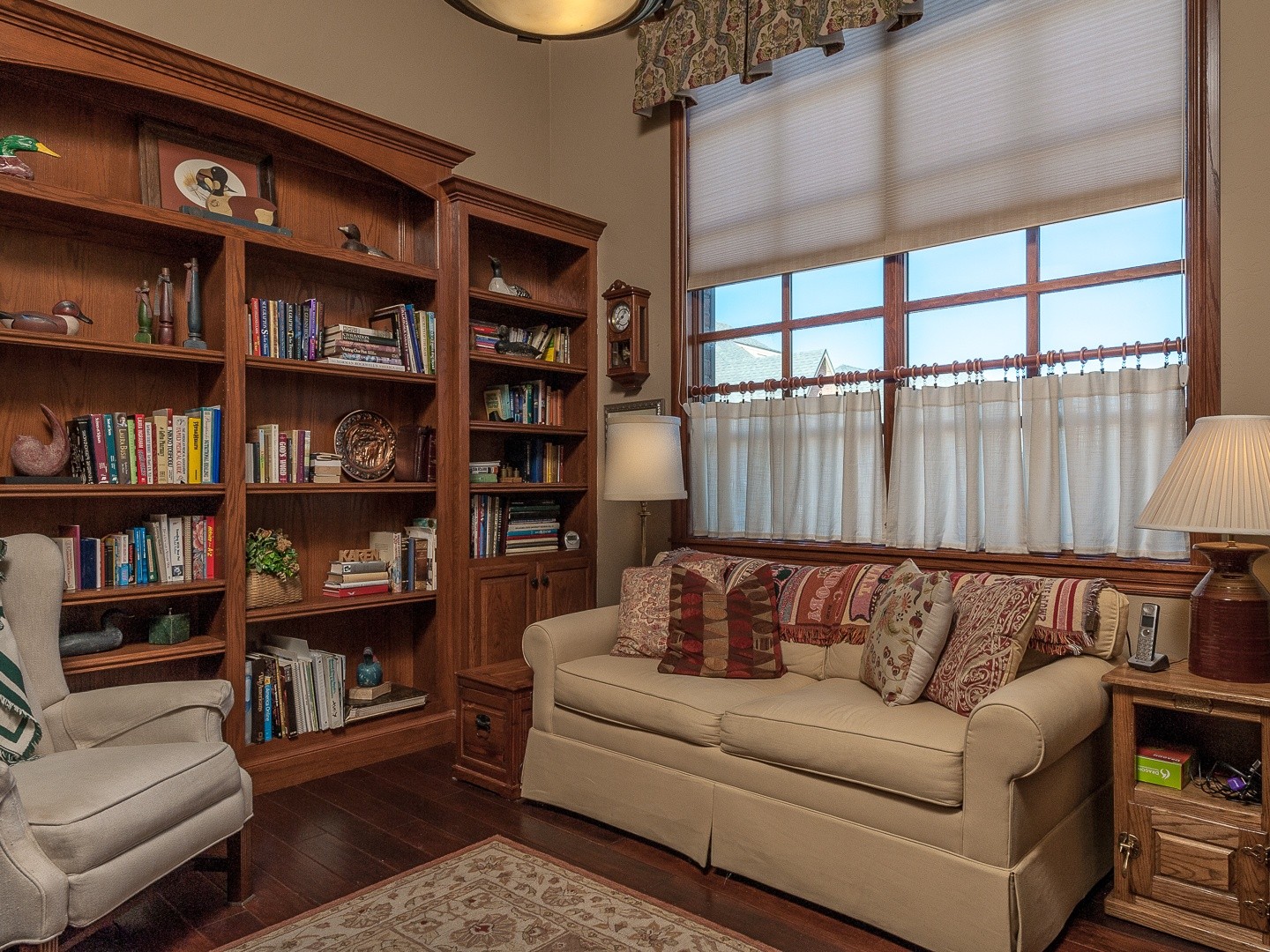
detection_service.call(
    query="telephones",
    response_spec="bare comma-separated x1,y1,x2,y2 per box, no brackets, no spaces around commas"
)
1127,603,1170,672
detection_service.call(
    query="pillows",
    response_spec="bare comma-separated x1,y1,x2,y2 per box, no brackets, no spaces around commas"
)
859,556,954,708
609,558,725,657
656,563,788,679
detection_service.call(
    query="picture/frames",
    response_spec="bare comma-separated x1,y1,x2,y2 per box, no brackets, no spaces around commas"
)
604,397,666,462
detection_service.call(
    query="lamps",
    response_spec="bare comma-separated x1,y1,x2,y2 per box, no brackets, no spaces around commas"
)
1133,414,1270,684
603,416,688,567
444,0,675,45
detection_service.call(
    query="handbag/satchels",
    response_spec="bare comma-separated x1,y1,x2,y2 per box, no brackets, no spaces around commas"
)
657,563,788,680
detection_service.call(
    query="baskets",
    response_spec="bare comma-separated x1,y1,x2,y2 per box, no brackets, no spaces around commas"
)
245,569,303,609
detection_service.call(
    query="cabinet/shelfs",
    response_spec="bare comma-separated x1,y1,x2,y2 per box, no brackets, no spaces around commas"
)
0,0,609,791
1101,656,1270,952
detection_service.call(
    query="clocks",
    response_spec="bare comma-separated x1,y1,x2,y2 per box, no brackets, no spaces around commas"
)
601,279,652,391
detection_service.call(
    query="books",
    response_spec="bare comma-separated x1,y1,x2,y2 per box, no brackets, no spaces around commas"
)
468,320,573,364
65,401,223,487
244,635,430,745
470,493,561,559
469,436,566,484
242,296,438,376
322,515,438,601
47,514,224,597
484,378,565,430
246,416,345,486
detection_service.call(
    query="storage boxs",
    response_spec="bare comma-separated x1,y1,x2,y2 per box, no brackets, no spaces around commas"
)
1134,736,1199,791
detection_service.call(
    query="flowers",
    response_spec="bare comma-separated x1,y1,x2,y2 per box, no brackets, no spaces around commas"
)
245,526,301,583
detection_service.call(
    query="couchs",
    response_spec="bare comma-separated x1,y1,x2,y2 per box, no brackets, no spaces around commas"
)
516,554,1129,951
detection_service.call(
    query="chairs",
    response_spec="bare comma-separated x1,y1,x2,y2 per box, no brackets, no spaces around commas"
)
0,533,256,951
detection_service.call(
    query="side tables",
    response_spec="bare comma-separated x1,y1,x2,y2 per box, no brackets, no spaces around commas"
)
452,653,534,803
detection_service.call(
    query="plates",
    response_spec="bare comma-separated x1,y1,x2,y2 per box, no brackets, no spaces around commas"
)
334,409,398,482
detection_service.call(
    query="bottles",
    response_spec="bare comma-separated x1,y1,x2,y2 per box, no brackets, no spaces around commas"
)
499,462,520,480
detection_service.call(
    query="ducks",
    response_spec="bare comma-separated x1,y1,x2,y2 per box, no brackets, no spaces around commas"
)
0,299,94,336
198,166,278,227
0,135,63,181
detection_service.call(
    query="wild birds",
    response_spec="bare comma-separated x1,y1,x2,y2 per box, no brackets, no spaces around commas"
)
494,325,544,359
488,254,531,300
338,223,395,259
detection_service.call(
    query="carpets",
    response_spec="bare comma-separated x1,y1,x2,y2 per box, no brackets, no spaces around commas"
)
204,834,778,952
